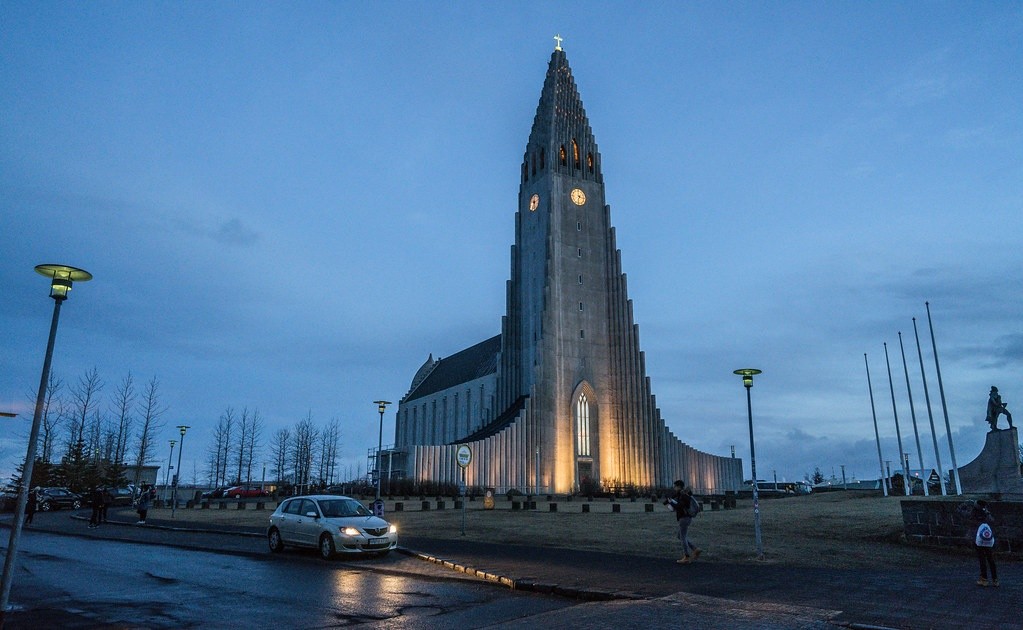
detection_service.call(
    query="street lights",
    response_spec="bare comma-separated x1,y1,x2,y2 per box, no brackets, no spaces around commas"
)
164,440,178,502
0,264,93,611
172,425,191,517
373,400,391,516
733,367,762,544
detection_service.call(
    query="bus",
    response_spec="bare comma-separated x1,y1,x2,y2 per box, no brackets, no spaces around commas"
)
757,481,812,493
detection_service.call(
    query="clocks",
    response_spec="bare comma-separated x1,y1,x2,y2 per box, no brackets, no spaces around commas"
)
528,194,540,211
571,189,586,205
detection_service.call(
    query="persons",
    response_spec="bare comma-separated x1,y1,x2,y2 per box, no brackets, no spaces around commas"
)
87,487,114,528
669,480,702,563
24,486,41,526
686,487,692,496
795,487,801,496
968,499,999,587
136,481,150,523
985,386,1016,431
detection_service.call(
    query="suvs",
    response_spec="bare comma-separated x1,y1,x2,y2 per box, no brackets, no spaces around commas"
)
31,488,82,512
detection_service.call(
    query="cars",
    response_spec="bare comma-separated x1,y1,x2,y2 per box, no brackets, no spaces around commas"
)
223,485,270,498
266,495,399,559
106,484,156,504
327,486,343,491
202,486,234,498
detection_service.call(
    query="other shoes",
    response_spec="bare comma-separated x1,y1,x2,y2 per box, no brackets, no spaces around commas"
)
137,521,145,524
977,578,990,587
677,554,692,564
990,579,999,587
692,548,702,560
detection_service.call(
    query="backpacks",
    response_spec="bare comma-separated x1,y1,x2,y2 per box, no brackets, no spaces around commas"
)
682,496,700,518
975,522,994,547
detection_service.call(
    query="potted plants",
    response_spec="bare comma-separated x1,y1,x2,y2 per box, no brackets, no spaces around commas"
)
506,488,522,501
385,477,480,501
585,482,659,502
